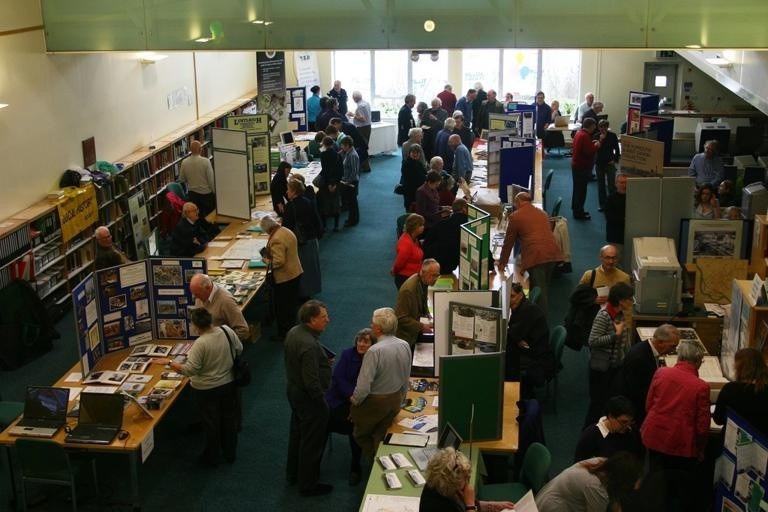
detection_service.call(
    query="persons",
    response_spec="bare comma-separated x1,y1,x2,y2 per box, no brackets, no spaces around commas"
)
171,307,244,470
260,78,767,509
189,273,251,433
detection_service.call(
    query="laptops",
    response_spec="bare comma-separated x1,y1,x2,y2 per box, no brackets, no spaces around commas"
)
69,392,122,444
10,385,67,436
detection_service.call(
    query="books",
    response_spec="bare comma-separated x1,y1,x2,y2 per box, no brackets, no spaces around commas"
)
1,98,272,309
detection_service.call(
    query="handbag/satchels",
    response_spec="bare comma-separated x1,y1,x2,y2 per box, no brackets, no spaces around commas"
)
232,350,251,386
262,272,275,302
589,350,609,371
313,175,327,187
394,183,406,195
563,324,587,352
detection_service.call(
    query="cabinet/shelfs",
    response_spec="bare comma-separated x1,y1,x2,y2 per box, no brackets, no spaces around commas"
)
153,118,218,234
215,96,257,130
1,152,159,308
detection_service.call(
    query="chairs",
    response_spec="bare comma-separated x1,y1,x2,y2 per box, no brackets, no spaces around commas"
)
548,197,563,219
481,443,549,505
12,437,100,509
543,324,568,399
543,169,554,203
528,287,541,304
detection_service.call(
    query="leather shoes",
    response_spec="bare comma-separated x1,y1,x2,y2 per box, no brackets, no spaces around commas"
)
573,211,591,220
588,173,597,182
348,463,362,486
300,483,333,497
322,225,339,233
344,217,359,227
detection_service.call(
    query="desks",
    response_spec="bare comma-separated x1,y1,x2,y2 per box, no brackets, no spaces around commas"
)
271,130,319,162
628,294,725,355
1,340,194,511
194,222,283,315
488,216,518,264
359,441,477,511
385,377,520,478
543,118,583,158
454,135,544,213
368,121,397,158
411,263,528,369
204,160,322,226
